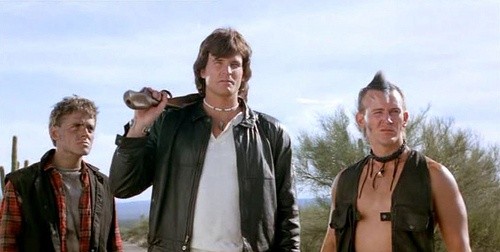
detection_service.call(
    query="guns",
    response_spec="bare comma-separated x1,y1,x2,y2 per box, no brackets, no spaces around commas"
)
124,87,205,111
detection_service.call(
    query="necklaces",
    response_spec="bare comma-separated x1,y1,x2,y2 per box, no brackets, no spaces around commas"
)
204,98,239,111
359,139,406,198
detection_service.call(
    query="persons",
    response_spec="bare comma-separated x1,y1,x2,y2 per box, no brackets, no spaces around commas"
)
0,94,123,252
109,28,301,252
321,73,471,252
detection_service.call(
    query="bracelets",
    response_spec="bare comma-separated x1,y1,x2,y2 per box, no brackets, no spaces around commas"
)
129,119,151,134
